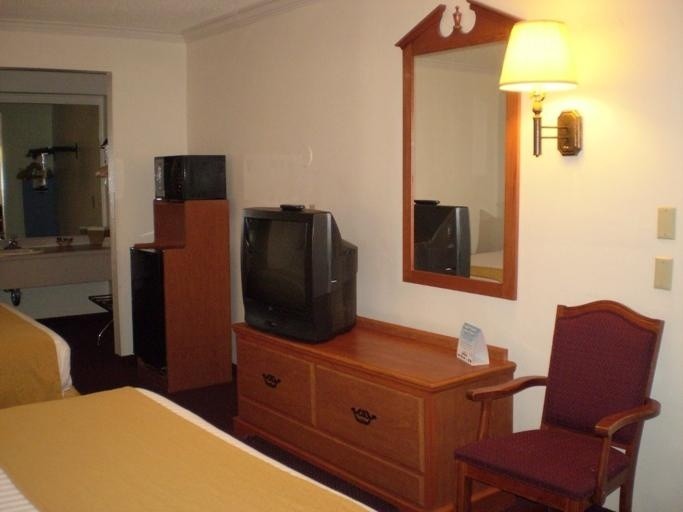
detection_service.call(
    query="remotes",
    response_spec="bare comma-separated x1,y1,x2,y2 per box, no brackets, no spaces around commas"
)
279,204,305,210
414,199,441,205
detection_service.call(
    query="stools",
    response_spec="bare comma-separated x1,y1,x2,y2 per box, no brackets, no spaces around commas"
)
88,293,113,346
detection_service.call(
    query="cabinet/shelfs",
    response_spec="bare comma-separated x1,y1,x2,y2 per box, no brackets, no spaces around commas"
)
230,315,516,512
130,199,233,394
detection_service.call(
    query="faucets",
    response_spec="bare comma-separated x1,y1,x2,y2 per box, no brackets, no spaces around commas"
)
4,241,22,249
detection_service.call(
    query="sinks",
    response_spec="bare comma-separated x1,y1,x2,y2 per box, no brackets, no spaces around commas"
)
0,247,46,256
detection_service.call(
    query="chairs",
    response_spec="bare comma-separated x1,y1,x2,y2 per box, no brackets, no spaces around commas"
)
453,299,663,512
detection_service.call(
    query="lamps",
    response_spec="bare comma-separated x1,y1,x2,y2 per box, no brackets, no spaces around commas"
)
496,19,582,156
25,144,79,192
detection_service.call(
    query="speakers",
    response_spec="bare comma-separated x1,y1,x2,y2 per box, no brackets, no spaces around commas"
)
164,155,226,200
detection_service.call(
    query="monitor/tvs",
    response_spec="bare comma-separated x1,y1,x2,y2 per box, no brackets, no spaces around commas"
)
240,206,358,345
414,204,471,279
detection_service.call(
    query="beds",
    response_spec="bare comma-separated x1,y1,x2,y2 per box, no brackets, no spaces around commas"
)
0,385,378,512
468,250,503,284
0,301,81,410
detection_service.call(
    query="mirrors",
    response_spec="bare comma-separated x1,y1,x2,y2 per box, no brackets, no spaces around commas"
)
0,103,103,240
393,0,525,299
